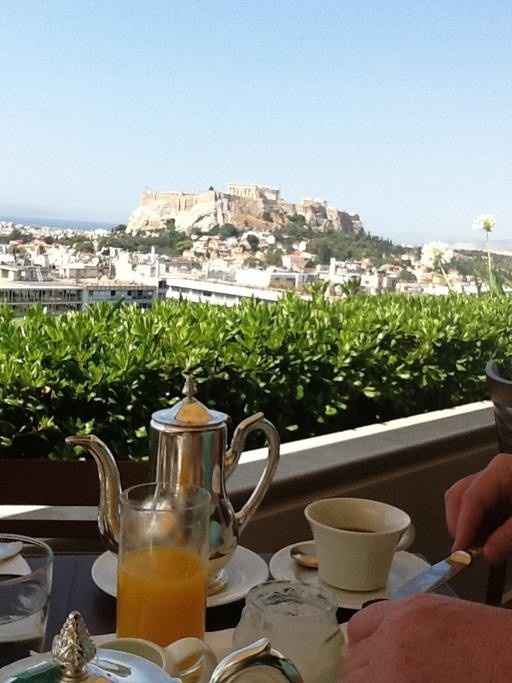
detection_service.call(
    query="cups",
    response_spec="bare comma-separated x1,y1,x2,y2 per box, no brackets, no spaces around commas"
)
302,497,413,592
1,533,55,667
232,580,346,683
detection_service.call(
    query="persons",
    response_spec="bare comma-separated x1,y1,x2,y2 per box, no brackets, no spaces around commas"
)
336,452,511,682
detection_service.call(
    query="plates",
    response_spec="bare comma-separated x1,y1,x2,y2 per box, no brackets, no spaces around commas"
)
91,543,270,608
269,538,434,612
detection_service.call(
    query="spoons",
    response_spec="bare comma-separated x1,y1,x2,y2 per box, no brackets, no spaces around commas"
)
290,540,317,570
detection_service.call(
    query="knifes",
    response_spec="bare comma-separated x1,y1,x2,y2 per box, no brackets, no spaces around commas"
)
386,546,486,599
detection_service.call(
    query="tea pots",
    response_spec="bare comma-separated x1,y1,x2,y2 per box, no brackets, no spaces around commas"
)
0,609,305,683
64,373,280,598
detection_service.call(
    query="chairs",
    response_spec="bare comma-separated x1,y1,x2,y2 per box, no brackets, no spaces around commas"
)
485,360,512,609
